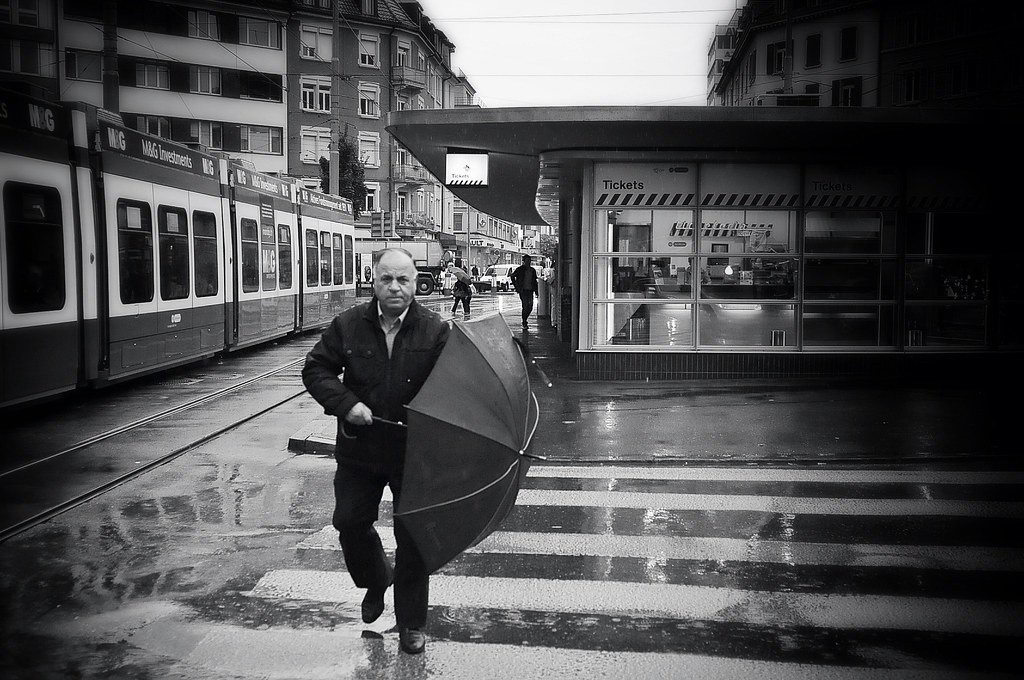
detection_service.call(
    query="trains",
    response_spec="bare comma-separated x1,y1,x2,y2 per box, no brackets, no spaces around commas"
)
1,89,359,409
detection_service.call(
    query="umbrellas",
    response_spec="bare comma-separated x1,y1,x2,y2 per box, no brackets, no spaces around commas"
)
451,267,472,287
370,312,547,576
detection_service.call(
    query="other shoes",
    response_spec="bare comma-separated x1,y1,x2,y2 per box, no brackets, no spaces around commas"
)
522,322,529,329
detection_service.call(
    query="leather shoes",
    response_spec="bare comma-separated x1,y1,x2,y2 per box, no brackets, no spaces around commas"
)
398,625,425,654
361,571,395,624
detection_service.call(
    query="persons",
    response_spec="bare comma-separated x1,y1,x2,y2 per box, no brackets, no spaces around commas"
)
471,265,479,277
301,248,451,654
686,257,712,285
451,279,472,314
511,254,538,329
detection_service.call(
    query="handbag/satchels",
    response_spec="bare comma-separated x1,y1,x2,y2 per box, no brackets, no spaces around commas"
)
455,290,465,298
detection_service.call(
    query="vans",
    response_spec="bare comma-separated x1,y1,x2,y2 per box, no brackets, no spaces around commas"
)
479,263,520,292
